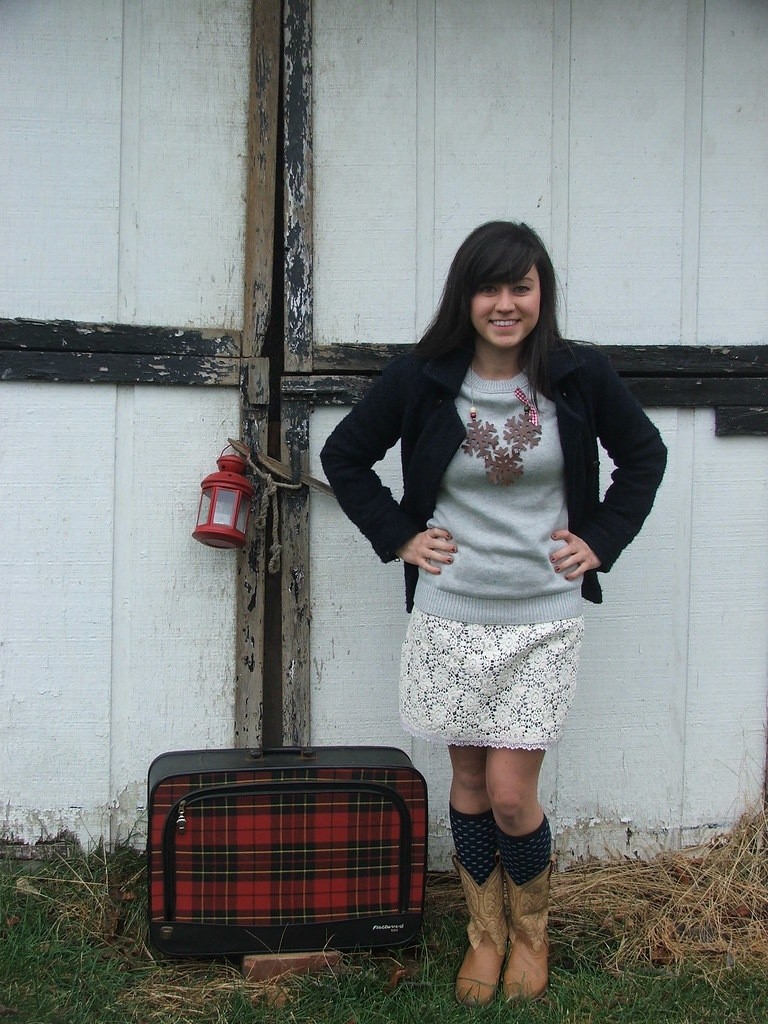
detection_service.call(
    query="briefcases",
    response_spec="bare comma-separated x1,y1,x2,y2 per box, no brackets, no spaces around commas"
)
142,745,430,966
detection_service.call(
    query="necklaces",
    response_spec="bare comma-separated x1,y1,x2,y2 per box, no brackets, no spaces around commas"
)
459,359,543,485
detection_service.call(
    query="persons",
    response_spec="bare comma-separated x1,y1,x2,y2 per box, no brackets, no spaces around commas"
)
319,221,668,1011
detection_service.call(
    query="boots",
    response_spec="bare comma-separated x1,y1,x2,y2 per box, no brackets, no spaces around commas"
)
500,860,554,1009
451,853,509,1012
